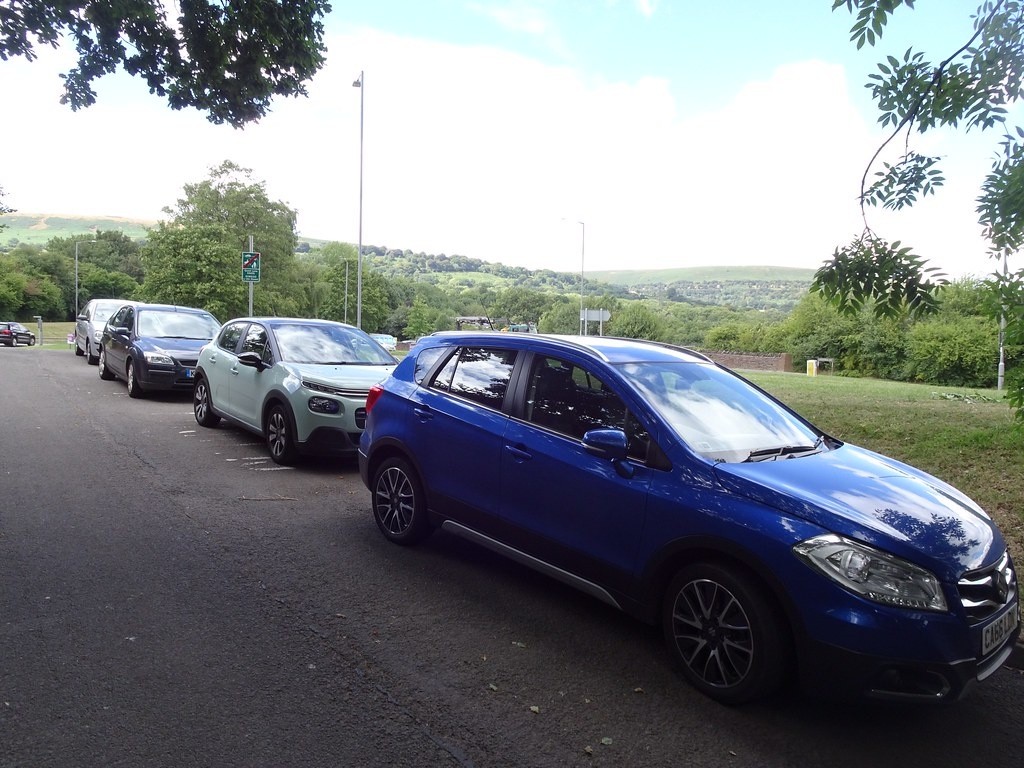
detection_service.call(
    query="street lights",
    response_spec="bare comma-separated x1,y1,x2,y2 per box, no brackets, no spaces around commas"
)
350,69,363,329
74,240,96,320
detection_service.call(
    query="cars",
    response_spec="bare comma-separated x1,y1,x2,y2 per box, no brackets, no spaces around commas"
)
0,321,35,347
356,330,1023,713
96,302,229,400
192,316,416,469
73,297,164,364
